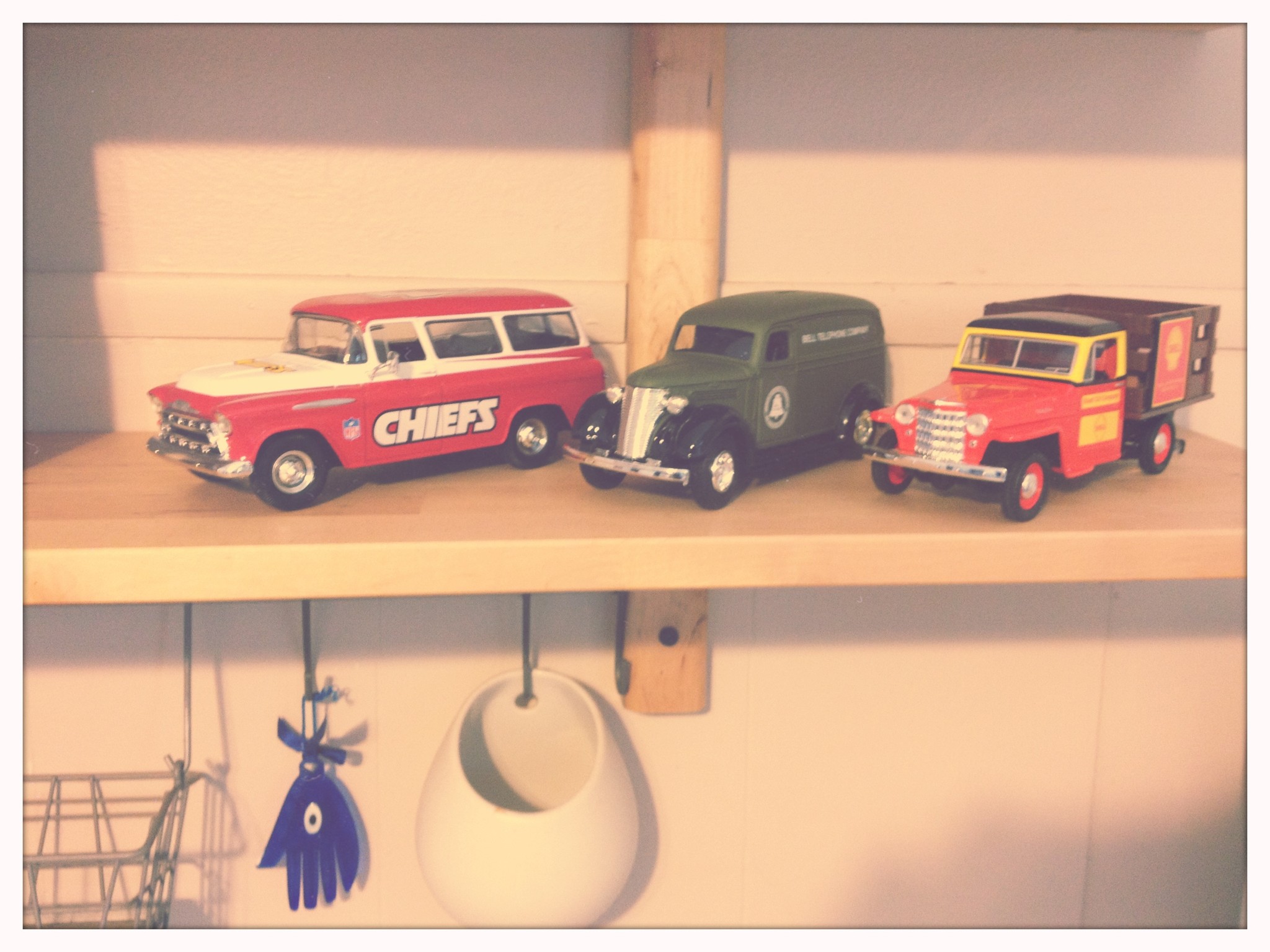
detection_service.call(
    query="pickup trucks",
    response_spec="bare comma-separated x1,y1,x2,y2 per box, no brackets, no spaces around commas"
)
861,293,1224,523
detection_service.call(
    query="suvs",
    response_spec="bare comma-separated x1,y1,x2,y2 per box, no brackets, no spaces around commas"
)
144,288,611,508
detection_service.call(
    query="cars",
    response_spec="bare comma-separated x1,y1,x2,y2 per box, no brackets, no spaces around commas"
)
561,289,893,512
